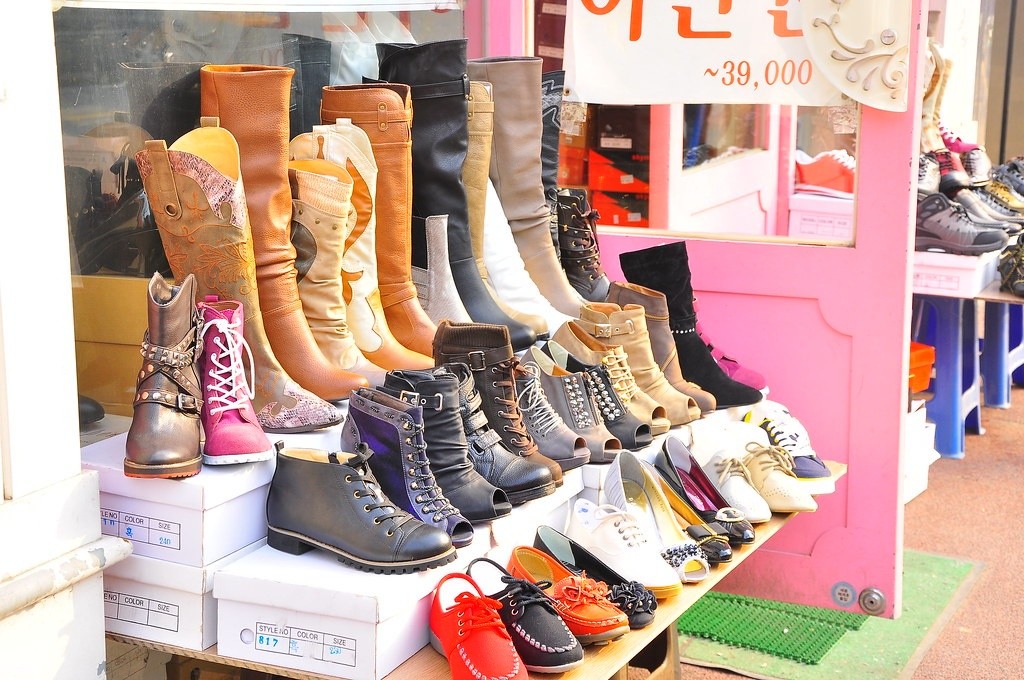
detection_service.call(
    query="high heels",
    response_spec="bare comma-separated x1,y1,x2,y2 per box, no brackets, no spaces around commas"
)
266,441,459,575
73,174,157,279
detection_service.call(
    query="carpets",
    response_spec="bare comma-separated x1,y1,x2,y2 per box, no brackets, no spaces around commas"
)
677,547,987,680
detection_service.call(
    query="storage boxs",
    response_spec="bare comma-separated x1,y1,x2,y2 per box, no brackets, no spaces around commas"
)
913,250,1001,299
75,339,142,416
787,193,854,242
212,523,491,680
102,536,268,652
909,343,935,394
577,424,693,505
80,398,349,568
70,273,175,346
486,466,585,568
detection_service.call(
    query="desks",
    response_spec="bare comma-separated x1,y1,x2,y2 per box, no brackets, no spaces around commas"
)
106,461,848,680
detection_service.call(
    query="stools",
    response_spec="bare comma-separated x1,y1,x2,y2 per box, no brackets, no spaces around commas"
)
922,300,1024,409
913,294,986,460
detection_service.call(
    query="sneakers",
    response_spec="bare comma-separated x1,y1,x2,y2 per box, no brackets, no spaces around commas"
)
917,146,1024,298
793,150,857,198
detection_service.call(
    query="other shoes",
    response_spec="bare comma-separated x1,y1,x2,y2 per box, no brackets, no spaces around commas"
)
79,391,104,427
428,404,837,680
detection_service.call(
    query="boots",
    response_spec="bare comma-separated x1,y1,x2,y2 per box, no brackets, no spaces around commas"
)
339,281,716,548
118,13,613,482
691,294,770,396
618,241,763,406
